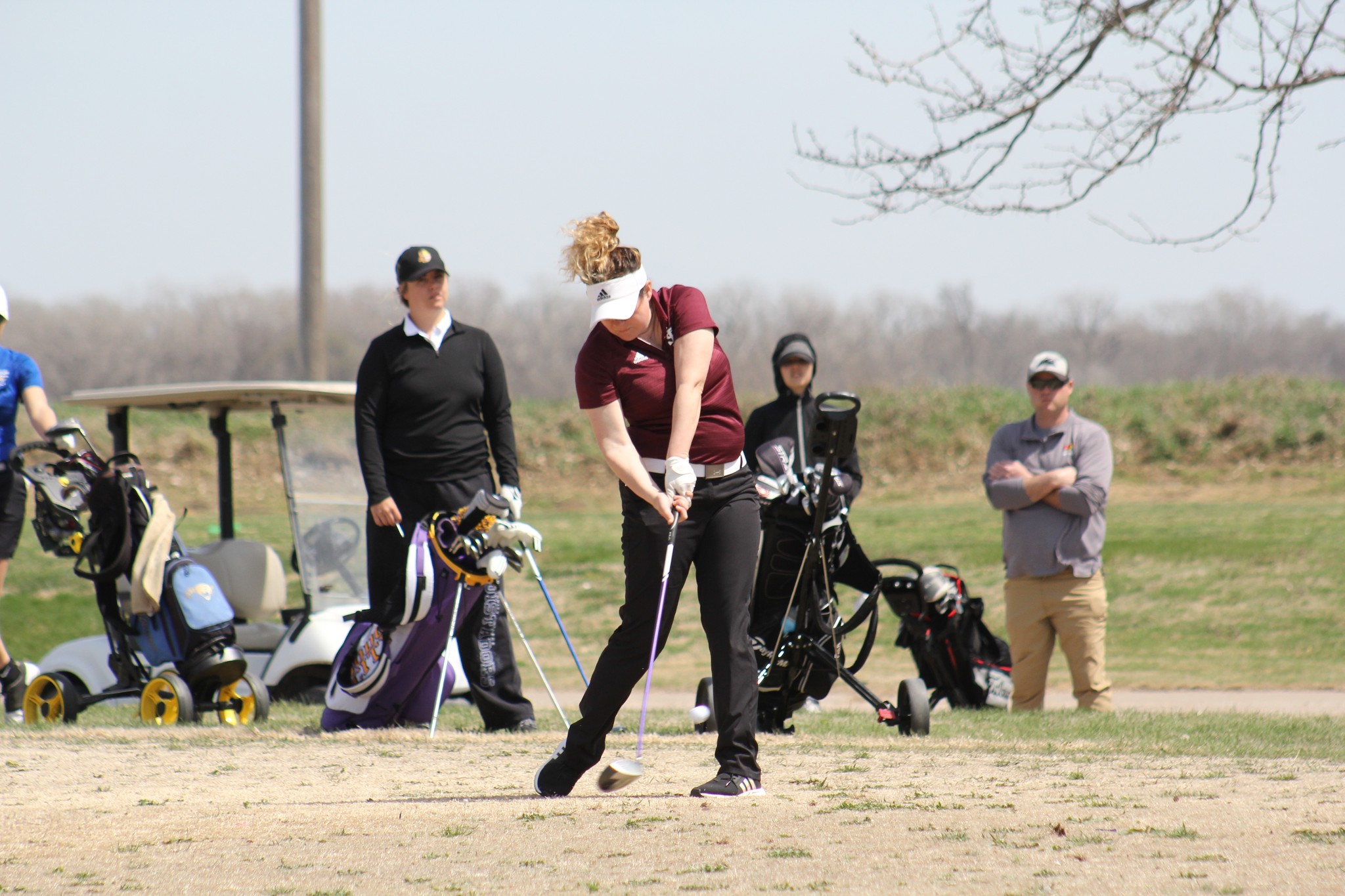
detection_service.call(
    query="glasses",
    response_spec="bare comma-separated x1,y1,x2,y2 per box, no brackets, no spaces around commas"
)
1028,378,1063,390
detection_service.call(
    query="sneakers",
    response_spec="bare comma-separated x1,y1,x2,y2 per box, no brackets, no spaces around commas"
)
690,772,766,799
803,698,824,716
1,660,39,726
534,738,575,795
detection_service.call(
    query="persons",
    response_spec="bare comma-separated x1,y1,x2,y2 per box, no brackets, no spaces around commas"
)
533,213,763,798
0,288,75,723
983,352,1113,713
354,245,540,731
740,335,862,716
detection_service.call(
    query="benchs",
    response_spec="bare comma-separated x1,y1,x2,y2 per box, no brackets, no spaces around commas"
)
183,539,290,650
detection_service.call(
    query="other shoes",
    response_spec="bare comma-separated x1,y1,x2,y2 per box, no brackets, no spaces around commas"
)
512,719,538,731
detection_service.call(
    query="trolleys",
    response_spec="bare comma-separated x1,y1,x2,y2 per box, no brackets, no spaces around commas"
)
693,389,929,737
871,558,1010,711
9,440,270,728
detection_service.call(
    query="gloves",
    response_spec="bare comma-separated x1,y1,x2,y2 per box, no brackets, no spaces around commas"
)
51,434,77,450
501,484,522,519
663,457,696,500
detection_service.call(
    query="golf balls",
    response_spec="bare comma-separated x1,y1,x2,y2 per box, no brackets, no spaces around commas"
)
686,705,711,725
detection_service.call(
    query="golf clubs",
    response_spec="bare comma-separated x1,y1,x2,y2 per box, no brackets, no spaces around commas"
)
43,417,104,462
457,531,522,570
784,453,841,510
504,510,591,692
597,492,680,793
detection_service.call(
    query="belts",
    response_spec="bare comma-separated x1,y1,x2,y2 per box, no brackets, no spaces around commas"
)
641,451,747,478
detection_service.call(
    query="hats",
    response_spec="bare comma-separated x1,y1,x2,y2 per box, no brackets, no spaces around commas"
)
395,247,450,281
777,339,814,363
0,286,10,321
1028,351,1070,382
586,267,651,329
